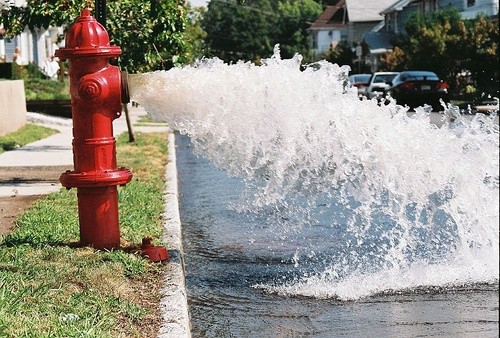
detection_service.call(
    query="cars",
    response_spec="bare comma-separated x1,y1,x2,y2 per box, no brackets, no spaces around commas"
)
383,71,448,111
350,74,372,93
365,72,400,99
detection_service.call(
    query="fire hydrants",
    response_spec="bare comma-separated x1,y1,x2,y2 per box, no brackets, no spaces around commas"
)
54,7,132,248
